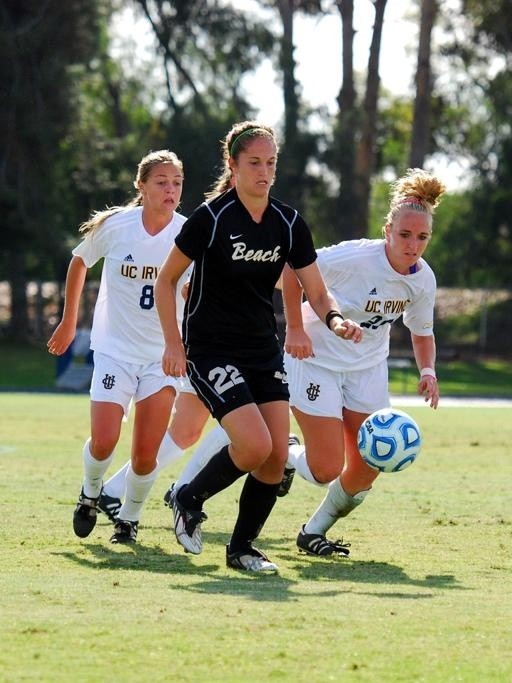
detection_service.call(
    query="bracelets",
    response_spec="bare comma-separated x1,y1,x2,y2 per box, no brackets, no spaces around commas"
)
325,310,344,330
420,367,438,380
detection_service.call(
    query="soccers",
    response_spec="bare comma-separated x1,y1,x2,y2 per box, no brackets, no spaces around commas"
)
357,408,423,473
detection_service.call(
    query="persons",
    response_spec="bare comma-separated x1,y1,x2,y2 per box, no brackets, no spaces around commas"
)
151,120,364,575
98,277,232,527
277,167,448,557
45,145,195,545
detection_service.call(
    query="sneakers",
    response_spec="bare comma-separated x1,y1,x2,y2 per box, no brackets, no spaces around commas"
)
296,524,349,556
276,434,300,497
226,544,278,571
96,486,121,522
73,481,104,537
163,483,208,520
172,484,202,554
110,517,139,544
